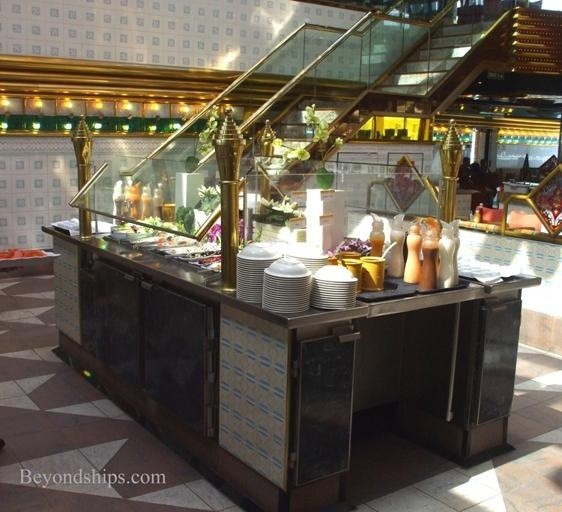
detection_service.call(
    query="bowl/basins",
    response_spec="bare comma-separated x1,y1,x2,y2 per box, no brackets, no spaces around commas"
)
236,243,358,316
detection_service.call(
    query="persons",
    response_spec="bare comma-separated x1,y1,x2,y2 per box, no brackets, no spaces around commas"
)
461,158,503,188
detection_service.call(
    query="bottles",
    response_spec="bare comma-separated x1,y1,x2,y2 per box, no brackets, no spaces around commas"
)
369,213,460,291
112,179,163,227
473,209,481,224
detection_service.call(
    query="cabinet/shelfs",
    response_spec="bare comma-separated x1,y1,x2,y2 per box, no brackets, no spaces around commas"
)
92,258,218,441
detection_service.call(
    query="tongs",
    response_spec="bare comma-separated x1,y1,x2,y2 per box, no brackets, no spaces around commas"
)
131,240,168,250
201,272,224,286
164,251,193,260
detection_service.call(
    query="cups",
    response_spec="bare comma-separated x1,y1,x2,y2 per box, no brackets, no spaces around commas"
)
337,249,387,292
162,204,176,223
